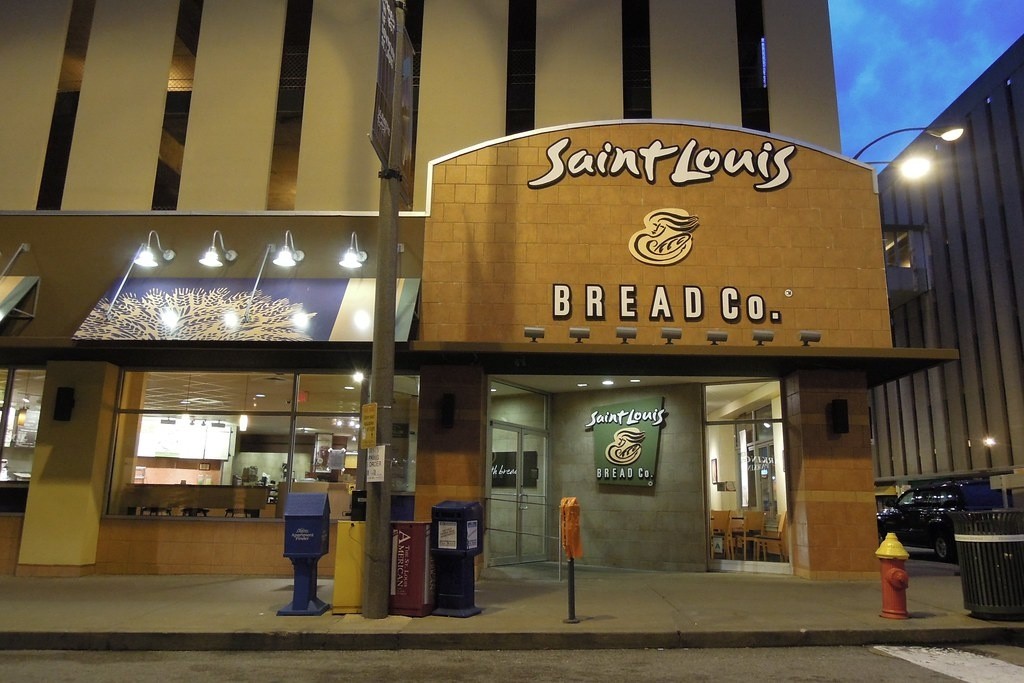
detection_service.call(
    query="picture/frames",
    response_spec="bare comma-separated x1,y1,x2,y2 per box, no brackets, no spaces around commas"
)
711,459,717,485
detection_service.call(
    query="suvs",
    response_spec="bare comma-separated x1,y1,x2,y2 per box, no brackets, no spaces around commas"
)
878,477,1012,565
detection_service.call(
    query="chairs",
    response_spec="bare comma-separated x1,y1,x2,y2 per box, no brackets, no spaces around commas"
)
711,509,788,564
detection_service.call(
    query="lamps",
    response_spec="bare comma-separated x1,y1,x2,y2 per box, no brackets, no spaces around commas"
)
339,232,367,268
751,330,774,346
660,327,683,345
239,374,248,431
135,230,176,267
202,420,206,426
190,420,194,425
831,399,849,434
17,373,29,425
853,127,966,160
615,327,637,344
569,327,590,343
706,331,728,345
798,333,821,346
523,327,545,343
273,230,305,267
200,230,238,267
212,420,225,428
161,417,175,424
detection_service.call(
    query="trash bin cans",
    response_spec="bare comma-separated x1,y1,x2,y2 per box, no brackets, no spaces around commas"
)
946,508,1024,622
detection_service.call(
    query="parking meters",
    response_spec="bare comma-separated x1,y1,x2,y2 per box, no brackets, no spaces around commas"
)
559,496,585,625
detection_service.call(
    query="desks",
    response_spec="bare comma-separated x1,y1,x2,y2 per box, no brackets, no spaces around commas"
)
127,484,270,518
711,518,753,560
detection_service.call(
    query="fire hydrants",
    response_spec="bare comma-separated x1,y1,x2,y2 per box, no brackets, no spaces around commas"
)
875,532,914,620
